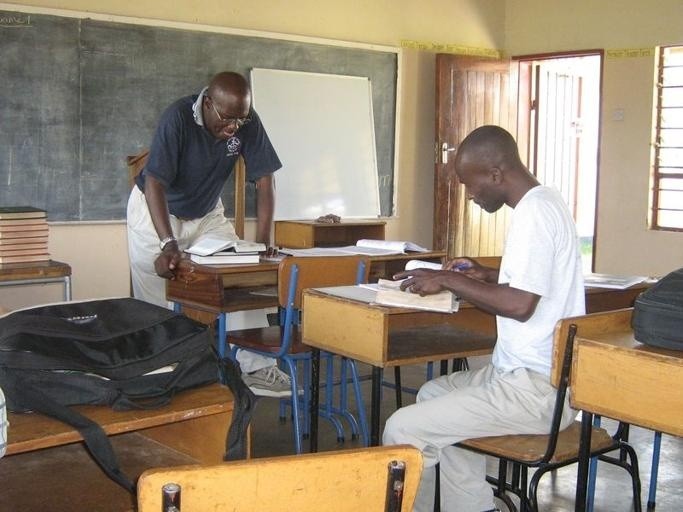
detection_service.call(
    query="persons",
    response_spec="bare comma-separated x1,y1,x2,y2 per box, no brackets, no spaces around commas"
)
127,72,283,309
382,126,586,512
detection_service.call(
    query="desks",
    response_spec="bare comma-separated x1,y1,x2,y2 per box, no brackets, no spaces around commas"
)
301,279,498,452
569,331,683,512
164,248,448,357
0,383,236,512
274,219,387,252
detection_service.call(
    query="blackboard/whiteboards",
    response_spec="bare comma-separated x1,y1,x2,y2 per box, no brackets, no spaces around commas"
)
0,2,402,226
248,66,382,224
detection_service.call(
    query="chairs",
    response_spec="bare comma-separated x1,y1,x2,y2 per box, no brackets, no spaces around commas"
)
432,307,644,512
139,442,424,512
227,253,371,458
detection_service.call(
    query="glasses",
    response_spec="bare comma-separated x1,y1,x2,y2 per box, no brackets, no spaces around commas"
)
210,100,255,125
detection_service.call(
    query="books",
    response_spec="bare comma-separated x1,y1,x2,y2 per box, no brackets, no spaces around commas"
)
183,235,267,264
334,238,433,256
0,206,51,264
375,259,461,314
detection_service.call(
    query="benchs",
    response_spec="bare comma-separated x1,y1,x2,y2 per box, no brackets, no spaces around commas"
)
0,210,72,302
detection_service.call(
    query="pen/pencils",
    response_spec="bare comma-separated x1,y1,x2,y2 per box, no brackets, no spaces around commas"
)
453,264,468,270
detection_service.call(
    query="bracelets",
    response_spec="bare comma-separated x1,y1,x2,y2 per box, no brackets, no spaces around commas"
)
159,235,177,250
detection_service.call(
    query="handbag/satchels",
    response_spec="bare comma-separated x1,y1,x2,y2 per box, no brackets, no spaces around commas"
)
0,296,258,500
629,266,683,354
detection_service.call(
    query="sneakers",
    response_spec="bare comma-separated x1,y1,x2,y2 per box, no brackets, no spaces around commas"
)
241,364,307,398
